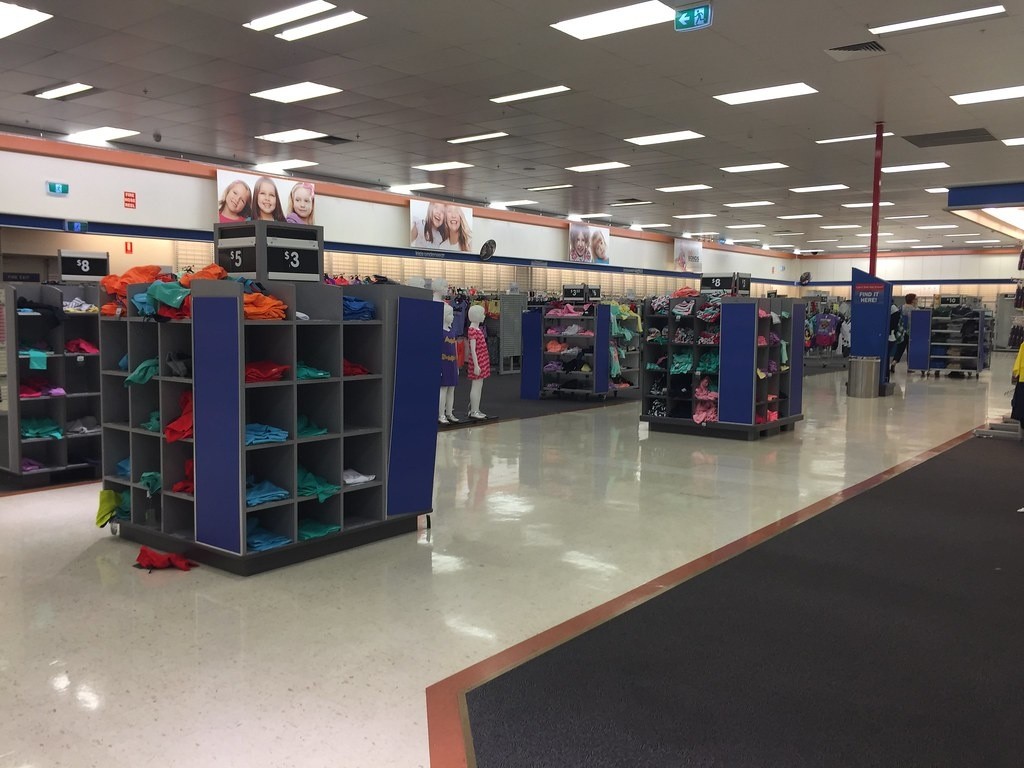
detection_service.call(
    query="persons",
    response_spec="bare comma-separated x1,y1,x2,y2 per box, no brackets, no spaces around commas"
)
590,231,609,264
569,223,591,263
465,304,491,419
408,275,426,288
410,201,471,251
891,292,920,373
438,302,462,425
217,179,253,223
285,181,315,225
431,277,448,301
246,176,288,222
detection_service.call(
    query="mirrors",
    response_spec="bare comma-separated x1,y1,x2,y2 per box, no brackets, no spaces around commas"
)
480,240,496,261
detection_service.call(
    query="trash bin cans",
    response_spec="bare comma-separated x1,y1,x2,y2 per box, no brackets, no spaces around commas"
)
848,355,881,399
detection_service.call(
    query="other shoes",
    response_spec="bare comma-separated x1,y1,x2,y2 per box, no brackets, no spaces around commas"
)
907,370,915,373
891,362,896,373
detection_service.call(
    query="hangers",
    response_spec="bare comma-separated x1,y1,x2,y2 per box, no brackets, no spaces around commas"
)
1012,317,1024,329
454,288,500,303
1017,280,1024,288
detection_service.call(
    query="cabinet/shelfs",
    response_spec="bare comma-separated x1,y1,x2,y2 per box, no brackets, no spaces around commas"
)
519,304,644,401
907,307,996,381
0,280,103,489
98,277,445,577
640,295,806,442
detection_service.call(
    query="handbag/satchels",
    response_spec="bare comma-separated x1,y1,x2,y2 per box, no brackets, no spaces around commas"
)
894,324,904,342
